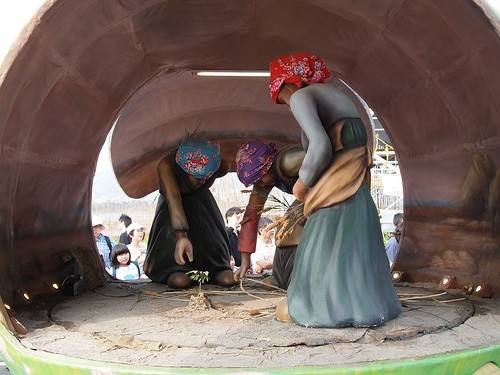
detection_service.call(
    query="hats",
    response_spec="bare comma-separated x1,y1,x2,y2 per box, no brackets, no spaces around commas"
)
126,222,146,237
92,219,105,230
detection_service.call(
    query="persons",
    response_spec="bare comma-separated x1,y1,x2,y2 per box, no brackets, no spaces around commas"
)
233,138,307,288
92,201,404,281
143,138,238,287
270,53,401,327
